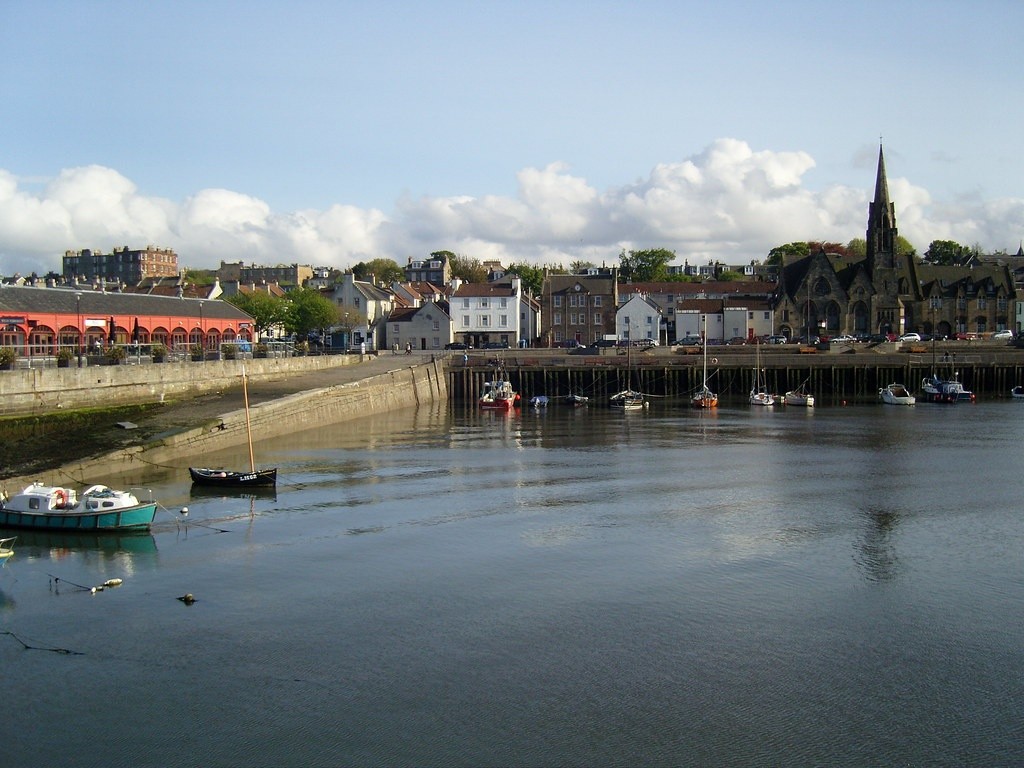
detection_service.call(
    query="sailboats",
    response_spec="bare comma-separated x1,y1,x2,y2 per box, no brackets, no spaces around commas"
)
691,314,719,408
609,321,643,411
188,364,277,487
749,339,774,405
921,302,973,402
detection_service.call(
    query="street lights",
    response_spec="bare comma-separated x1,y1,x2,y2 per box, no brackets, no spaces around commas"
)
199,301,205,358
283,306,289,357
344,312,349,355
74,293,83,368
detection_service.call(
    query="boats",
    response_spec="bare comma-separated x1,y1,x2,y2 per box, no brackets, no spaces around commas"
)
880,383,915,405
0,536,16,568
531,396,549,407
565,395,588,407
0,480,158,533
786,391,814,406
773,395,785,404
480,382,517,410
1011,386,1024,398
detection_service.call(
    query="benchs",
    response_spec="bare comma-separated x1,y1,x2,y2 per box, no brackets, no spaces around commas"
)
911,345,927,353
686,348,700,354
799,347,816,354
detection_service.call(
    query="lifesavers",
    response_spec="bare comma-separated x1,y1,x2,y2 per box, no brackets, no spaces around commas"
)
760,396,764,399
56,490,66,507
712,358,718,364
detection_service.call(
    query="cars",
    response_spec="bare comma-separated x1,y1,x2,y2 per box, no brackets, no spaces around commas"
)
259,333,330,347
723,329,1024,346
555,338,587,348
445,342,472,350
590,338,659,347
483,342,508,349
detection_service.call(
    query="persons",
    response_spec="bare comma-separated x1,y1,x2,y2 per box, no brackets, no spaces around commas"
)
93,337,105,354
392,341,413,356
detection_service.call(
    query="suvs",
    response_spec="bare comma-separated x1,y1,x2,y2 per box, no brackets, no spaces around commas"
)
672,334,703,346
